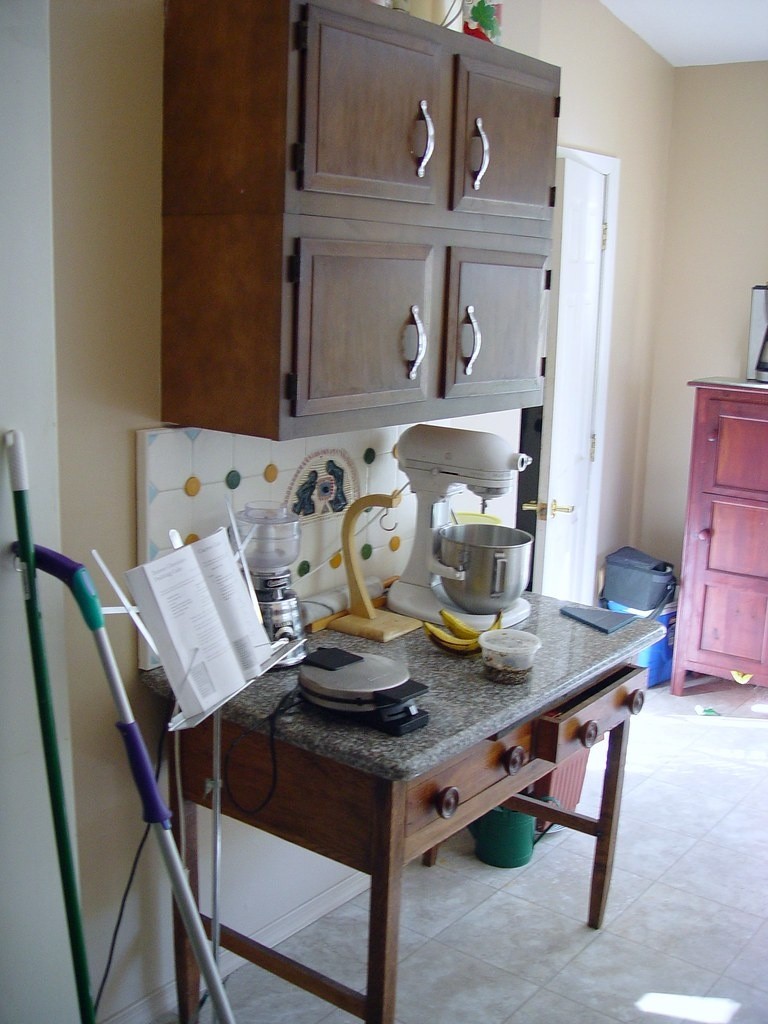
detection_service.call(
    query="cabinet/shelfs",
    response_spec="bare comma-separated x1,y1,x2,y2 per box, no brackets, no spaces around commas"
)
672,376,768,696
160,0,562,441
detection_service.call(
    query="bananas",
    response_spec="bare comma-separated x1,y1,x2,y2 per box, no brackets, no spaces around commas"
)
423,607,503,659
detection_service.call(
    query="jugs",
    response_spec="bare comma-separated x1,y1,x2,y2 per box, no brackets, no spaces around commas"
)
468,796,560,868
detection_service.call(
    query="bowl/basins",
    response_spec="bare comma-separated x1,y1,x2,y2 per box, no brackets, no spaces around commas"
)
480,628,542,685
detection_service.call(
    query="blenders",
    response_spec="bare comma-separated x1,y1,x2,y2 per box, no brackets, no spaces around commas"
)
387,423,534,632
228,502,308,667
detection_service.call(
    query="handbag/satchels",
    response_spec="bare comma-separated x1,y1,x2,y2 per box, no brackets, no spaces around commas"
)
602,546,676,611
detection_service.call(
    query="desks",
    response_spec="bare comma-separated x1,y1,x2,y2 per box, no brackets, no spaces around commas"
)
139,593,668,1024
